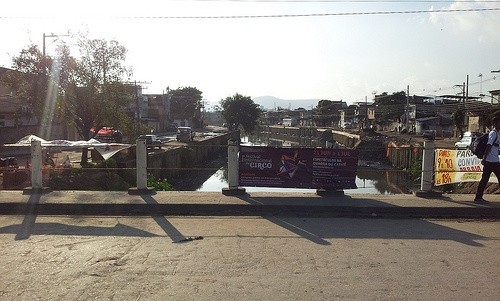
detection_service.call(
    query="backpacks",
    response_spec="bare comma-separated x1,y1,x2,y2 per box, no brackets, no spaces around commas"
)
469,130,498,159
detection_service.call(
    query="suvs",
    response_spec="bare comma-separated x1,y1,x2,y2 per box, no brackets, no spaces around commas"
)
176,127,196,140
139,134,161,149
453,131,482,151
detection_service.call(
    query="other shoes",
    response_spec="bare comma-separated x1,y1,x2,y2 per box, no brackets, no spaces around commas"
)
474,198,490,205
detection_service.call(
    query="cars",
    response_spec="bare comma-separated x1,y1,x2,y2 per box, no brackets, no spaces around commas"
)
274,119,391,133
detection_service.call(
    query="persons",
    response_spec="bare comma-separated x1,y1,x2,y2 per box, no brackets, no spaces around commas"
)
473,116,500,205
